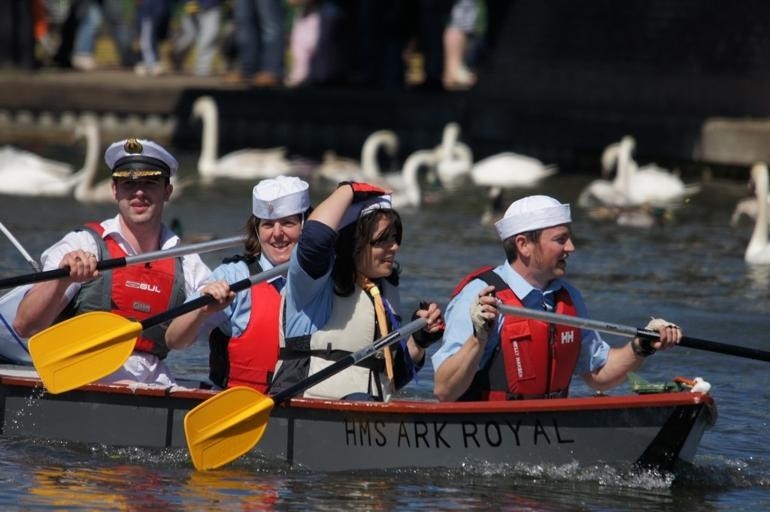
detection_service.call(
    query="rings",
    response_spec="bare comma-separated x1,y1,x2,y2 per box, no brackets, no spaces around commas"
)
74,256,82,263
88,253,97,260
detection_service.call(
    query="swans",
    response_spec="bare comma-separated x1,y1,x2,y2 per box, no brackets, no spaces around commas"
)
69,111,194,207
313,121,562,211
576,135,702,222
1,145,83,199
730,162,770,265
190,96,317,183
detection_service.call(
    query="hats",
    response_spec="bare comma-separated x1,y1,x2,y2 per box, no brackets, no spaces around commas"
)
494,194,573,241
335,193,394,231
251,174,311,221
103,137,179,181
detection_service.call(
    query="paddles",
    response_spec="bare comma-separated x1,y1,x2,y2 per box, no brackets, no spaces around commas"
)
28,261,290,395
184,316,428,473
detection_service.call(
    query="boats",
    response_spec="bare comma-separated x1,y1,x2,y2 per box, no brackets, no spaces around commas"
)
0,367,718,473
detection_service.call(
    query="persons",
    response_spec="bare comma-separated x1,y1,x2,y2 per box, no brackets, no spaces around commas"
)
263,178,447,404
430,193,683,403
11,140,215,386
162,171,316,396
